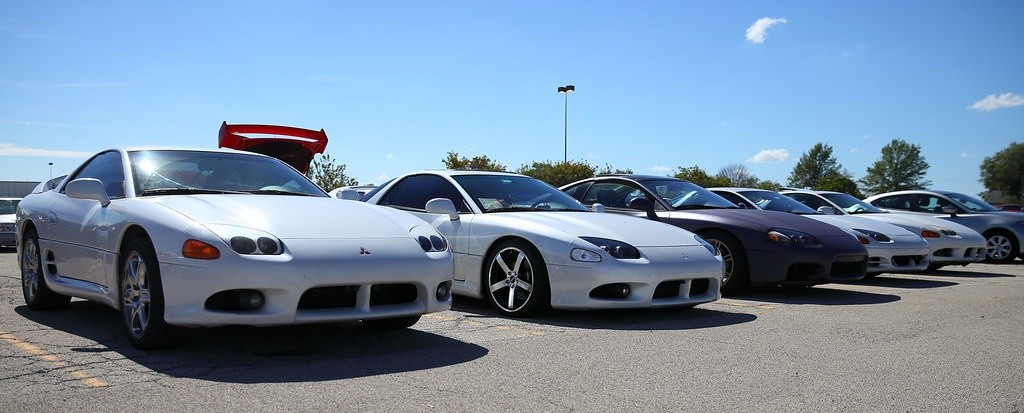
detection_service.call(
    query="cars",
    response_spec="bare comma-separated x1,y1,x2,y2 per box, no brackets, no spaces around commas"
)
16,146,456,349
750,191,928,279
359,171,725,321
862,190,1024,261
510,175,869,290
0,196,24,249
779,186,987,272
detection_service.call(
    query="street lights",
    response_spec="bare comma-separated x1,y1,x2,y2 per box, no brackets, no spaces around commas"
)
558,82,573,159
